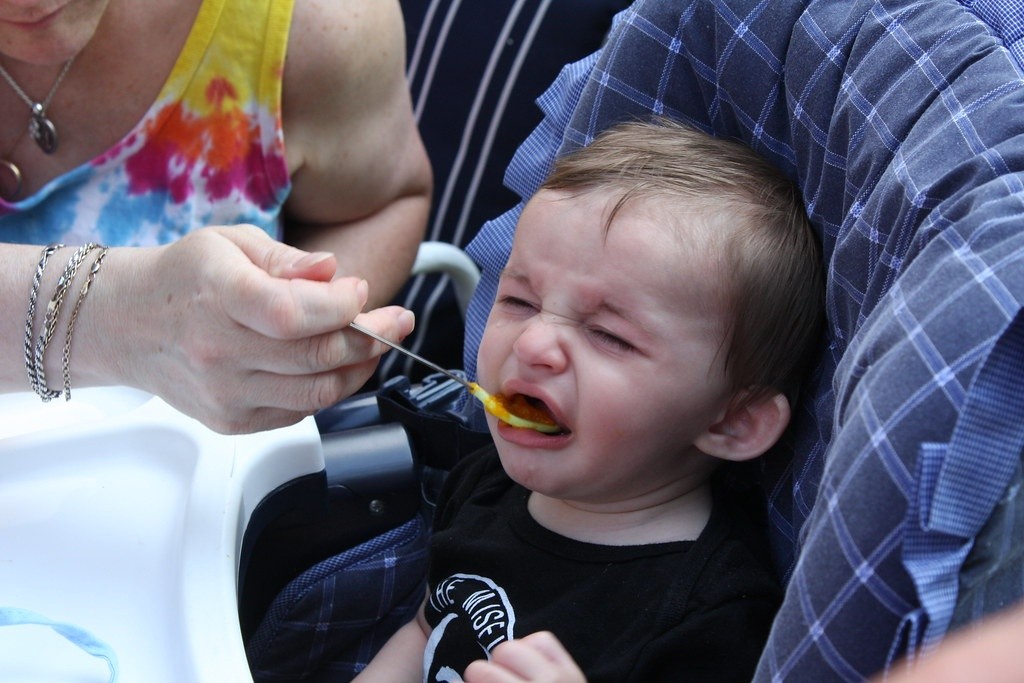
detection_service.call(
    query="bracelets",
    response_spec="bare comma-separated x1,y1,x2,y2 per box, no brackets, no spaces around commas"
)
23,242,109,404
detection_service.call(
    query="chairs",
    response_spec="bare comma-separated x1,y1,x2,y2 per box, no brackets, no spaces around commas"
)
210,0,1022,681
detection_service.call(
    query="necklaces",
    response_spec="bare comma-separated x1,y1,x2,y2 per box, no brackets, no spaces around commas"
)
0,61,74,199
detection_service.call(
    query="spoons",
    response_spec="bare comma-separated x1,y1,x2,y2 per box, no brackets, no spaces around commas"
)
348,319,561,432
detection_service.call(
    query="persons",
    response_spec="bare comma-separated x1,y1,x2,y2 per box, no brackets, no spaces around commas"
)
0,0,436,683
342,116,825,683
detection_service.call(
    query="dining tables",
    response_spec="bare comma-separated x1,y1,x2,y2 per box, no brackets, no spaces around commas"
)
1,384,328,681
